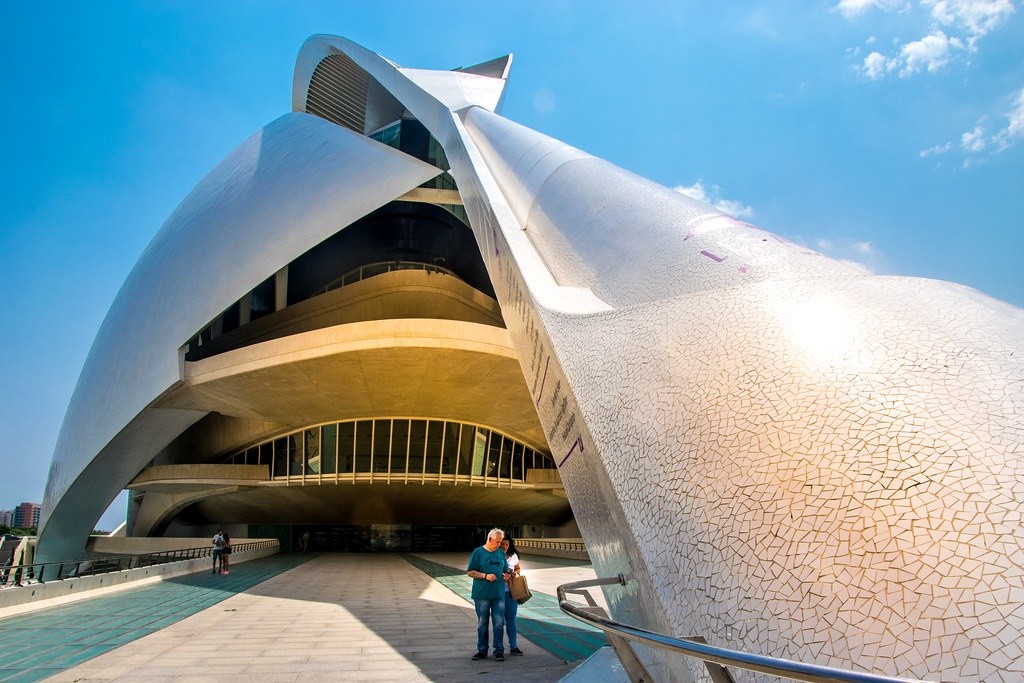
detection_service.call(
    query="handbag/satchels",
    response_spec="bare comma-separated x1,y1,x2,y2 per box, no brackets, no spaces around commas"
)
517,590,532,605
509,572,530,600
224,546,232,554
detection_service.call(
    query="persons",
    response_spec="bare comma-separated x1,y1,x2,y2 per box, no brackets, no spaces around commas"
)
302,530,310,554
467,529,511,661
220,533,231,574
492,538,531,656
212,529,223,574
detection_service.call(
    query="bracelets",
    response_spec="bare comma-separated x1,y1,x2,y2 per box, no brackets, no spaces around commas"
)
485,573,488,579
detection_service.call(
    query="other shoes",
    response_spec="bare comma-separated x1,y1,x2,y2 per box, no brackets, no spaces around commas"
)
212,570,216,573
219,570,221,573
223,571,229,574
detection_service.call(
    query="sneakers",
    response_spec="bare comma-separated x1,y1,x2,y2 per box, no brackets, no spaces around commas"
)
510,647,524,655
472,652,488,659
493,650,496,656
496,653,504,661
